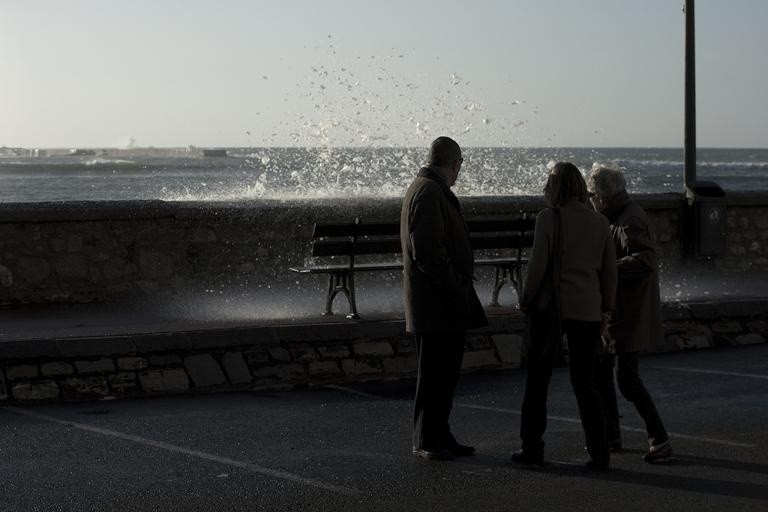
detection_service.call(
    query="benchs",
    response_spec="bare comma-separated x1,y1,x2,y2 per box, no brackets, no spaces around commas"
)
292,214,536,320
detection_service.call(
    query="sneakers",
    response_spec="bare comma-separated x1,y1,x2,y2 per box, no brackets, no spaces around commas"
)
510,446,546,468
641,441,676,463
581,434,625,473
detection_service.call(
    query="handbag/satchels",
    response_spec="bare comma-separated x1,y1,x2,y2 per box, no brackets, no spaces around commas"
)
518,206,565,317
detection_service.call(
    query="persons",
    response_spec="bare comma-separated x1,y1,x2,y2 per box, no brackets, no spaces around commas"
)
581,168,675,468
398,136,489,462
508,161,622,468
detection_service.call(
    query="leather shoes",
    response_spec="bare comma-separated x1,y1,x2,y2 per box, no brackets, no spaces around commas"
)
445,440,475,455
412,441,445,463
